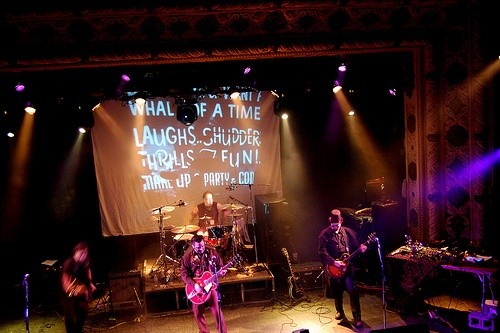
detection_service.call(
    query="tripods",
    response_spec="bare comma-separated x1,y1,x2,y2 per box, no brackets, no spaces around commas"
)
148,202,186,284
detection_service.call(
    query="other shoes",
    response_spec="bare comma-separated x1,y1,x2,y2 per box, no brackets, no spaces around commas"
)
354,319,363,327
334,313,345,319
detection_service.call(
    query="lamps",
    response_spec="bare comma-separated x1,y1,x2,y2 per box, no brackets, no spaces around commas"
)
177,105,198,125
274,99,291,118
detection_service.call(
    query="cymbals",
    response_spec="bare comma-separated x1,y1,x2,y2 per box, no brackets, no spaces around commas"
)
171,225,200,233
219,204,244,210
227,214,243,216
147,206,175,214
149,215,172,221
198,217,213,219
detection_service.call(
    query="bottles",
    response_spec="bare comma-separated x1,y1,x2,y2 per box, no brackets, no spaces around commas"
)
154,274,158,286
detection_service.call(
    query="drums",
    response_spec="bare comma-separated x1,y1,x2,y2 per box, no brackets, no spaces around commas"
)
173,225,232,268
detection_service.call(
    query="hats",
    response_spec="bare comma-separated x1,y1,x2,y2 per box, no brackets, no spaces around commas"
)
328,209,344,223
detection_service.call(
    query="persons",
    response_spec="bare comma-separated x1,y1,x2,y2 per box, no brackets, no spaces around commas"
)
61,243,96,333
181,235,228,333
188,190,230,233
318,209,367,328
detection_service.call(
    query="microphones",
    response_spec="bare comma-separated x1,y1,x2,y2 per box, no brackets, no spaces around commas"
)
225,186,237,190
176,202,188,206
212,256,216,264
374,238,381,247
23,274,30,282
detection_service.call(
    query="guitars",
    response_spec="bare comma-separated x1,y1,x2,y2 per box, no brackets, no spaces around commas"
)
280,247,304,301
326,232,377,280
185,254,243,305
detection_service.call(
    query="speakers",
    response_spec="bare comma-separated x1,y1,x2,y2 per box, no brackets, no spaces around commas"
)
292,261,326,290
108,271,143,310
